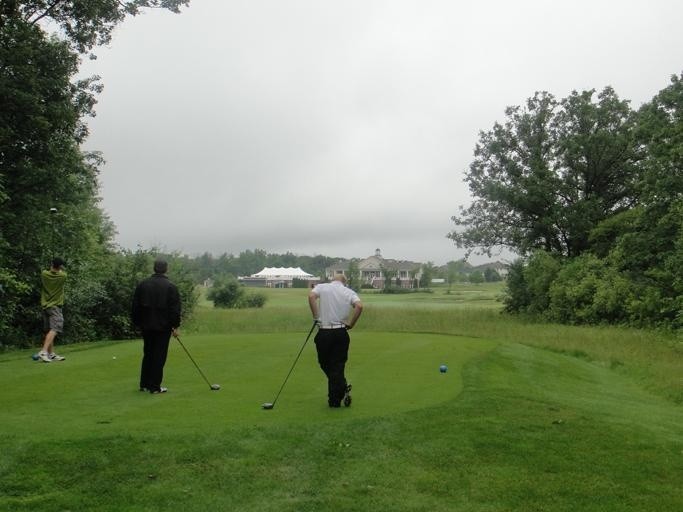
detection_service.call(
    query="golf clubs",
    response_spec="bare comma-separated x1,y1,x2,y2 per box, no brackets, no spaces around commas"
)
171,327,221,390
50,207,58,264
262,319,318,409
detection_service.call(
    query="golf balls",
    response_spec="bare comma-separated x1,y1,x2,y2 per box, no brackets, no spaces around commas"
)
113,356,116,360
32,354,39,360
440,365,447,373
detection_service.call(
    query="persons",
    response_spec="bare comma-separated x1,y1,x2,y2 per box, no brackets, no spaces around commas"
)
307,273,363,408
131,259,181,393
31,257,67,362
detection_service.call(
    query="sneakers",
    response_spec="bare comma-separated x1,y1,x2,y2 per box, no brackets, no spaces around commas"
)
344,383,352,407
140,387,168,394
38,350,65,362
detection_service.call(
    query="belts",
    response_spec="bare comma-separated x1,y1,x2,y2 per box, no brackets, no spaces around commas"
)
319,324,346,329
